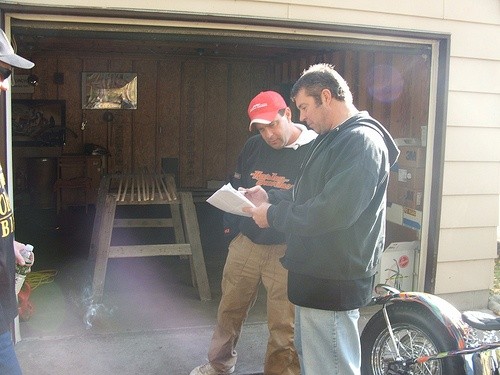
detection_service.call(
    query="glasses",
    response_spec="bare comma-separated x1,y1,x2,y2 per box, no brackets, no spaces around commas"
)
0,66,12,78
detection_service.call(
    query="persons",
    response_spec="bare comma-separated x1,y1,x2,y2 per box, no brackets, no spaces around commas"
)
0,28,35,375
241,63,400,375
189,91,319,375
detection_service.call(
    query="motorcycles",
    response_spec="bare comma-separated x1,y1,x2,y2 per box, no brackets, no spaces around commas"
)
360,284,500,375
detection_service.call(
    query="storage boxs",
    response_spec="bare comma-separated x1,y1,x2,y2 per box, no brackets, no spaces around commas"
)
372,242,417,297
387,145,426,210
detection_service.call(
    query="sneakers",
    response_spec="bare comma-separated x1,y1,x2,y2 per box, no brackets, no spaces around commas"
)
189,358,235,375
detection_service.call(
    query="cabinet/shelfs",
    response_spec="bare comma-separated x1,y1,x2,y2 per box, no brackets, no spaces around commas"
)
58,152,107,216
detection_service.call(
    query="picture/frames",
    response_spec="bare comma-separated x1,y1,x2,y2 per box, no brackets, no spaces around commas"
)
81,71,138,111
12,99,66,147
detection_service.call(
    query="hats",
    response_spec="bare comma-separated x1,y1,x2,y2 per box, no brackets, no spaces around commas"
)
247,91,287,132
0,27,35,70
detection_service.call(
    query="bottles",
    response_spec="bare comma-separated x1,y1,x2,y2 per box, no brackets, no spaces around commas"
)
15,245,34,293
